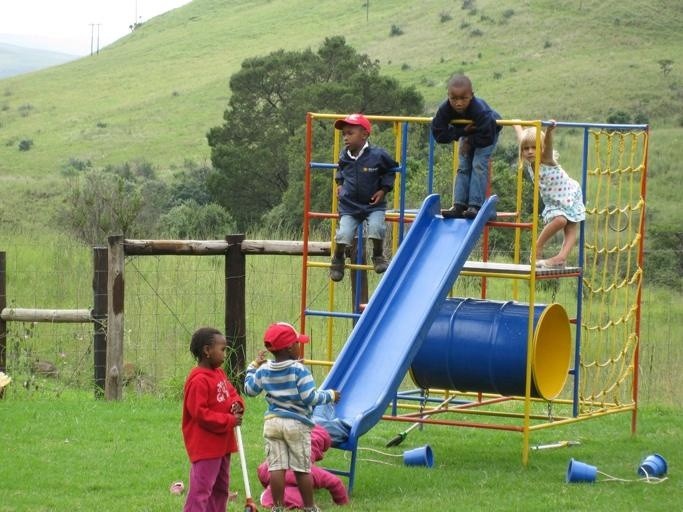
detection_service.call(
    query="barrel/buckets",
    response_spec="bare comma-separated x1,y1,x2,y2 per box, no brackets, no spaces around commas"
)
408,296,573,402
404,444,433,468
638,453,667,477
565,458,597,484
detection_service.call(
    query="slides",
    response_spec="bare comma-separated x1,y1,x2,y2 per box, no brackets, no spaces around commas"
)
306,193,497,448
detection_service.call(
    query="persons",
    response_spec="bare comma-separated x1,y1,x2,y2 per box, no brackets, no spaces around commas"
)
244,322,341,512
257,399,356,509
182,328,245,512
330,113,399,281
431,73,504,220
511,117,585,267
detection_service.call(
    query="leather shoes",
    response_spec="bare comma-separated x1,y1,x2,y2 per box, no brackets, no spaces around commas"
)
529,247,566,269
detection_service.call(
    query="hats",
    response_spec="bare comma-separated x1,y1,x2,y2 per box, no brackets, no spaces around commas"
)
334,113,371,136
263,322,310,352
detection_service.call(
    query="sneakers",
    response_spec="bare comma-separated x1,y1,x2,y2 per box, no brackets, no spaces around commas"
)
371,253,389,274
441,204,480,219
330,254,344,281
